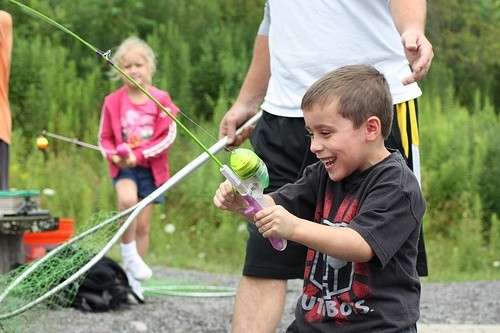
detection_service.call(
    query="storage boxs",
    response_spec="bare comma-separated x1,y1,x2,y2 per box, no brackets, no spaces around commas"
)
0,189,42,215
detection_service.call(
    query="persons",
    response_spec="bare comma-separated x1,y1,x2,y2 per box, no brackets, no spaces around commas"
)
0,11,15,193
218,0,434,333
212,63,427,333
97,37,179,300
